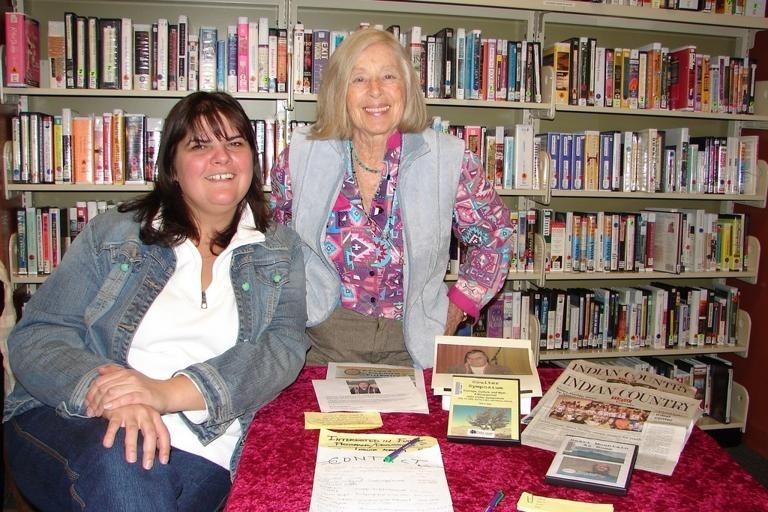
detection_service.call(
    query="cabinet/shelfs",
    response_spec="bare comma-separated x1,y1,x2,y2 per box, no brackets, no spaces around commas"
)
529,12,766,432
287,0,544,366
2,0,291,389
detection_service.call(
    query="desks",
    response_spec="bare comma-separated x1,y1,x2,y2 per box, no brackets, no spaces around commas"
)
222,364,768,512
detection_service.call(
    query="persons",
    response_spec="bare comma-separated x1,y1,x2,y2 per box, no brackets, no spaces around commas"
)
270,29,514,371
350,381,381,394
549,401,649,431
446,350,515,375
0,91,311,511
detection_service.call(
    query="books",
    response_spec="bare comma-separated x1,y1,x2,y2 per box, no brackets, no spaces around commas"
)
292,116,759,197
541,433,638,497
293,18,756,116
17,201,124,276
447,228,469,275
1,16,286,185
538,353,734,425
447,374,521,443
454,289,513,339
510,282,740,351
510,207,744,275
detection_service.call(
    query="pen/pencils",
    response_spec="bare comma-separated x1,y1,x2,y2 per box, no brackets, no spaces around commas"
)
383,437,420,463
484,490,504,512
